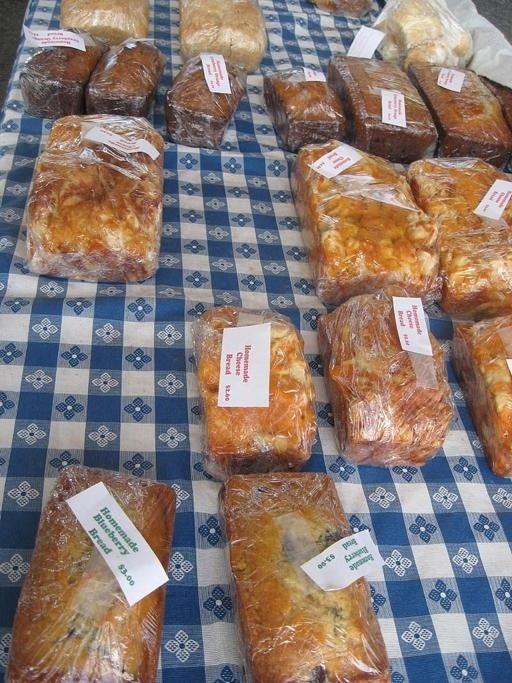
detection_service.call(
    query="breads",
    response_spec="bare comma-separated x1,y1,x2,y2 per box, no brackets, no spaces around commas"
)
224,471,391,683
20,27,247,148
292,139,512,317
264,54,511,168
56,1,149,46
197,306,318,478
27,114,163,282
450,314,512,477
6,464,177,683
318,286,454,468
179,1,267,74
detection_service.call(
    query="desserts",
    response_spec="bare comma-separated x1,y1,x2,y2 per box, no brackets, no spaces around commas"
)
373,1,472,71
